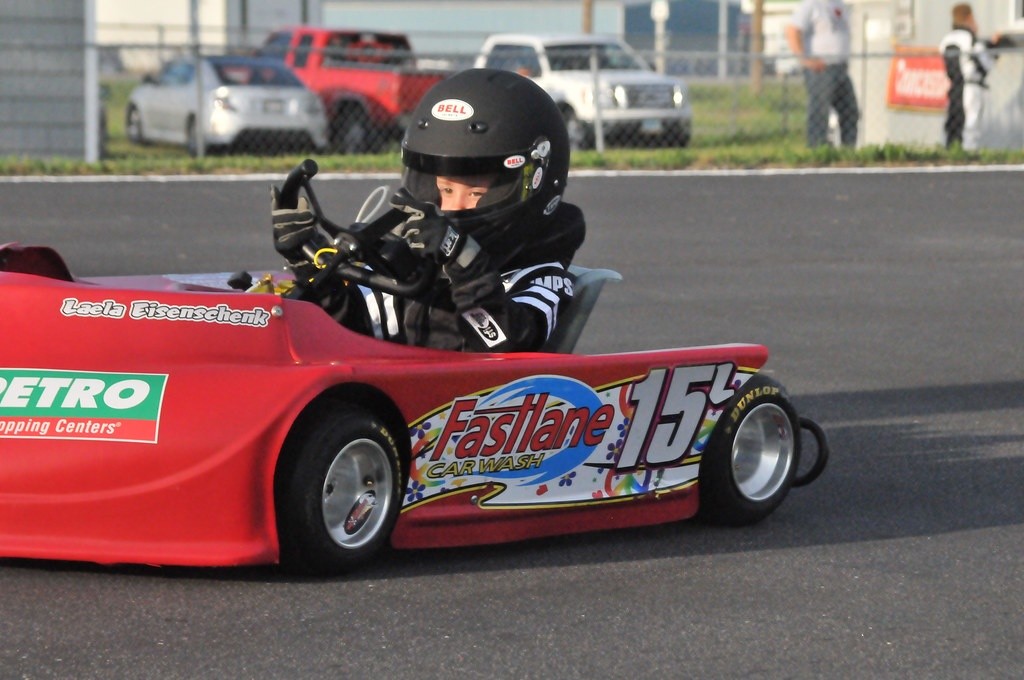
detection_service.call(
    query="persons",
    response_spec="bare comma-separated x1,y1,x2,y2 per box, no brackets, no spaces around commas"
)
941,5,1003,164
789,0,859,149
271,68,574,354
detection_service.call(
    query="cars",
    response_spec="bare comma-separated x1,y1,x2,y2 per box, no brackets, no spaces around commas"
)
473,34,692,150
124,56,330,160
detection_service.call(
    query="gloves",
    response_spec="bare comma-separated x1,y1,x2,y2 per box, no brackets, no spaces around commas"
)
389,187,495,288
270,184,332,290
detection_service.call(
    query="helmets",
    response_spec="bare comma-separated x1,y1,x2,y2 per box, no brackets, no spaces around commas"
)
388,68,570,269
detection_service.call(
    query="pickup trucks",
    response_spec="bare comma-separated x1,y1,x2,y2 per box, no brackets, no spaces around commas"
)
224,27,453,156
